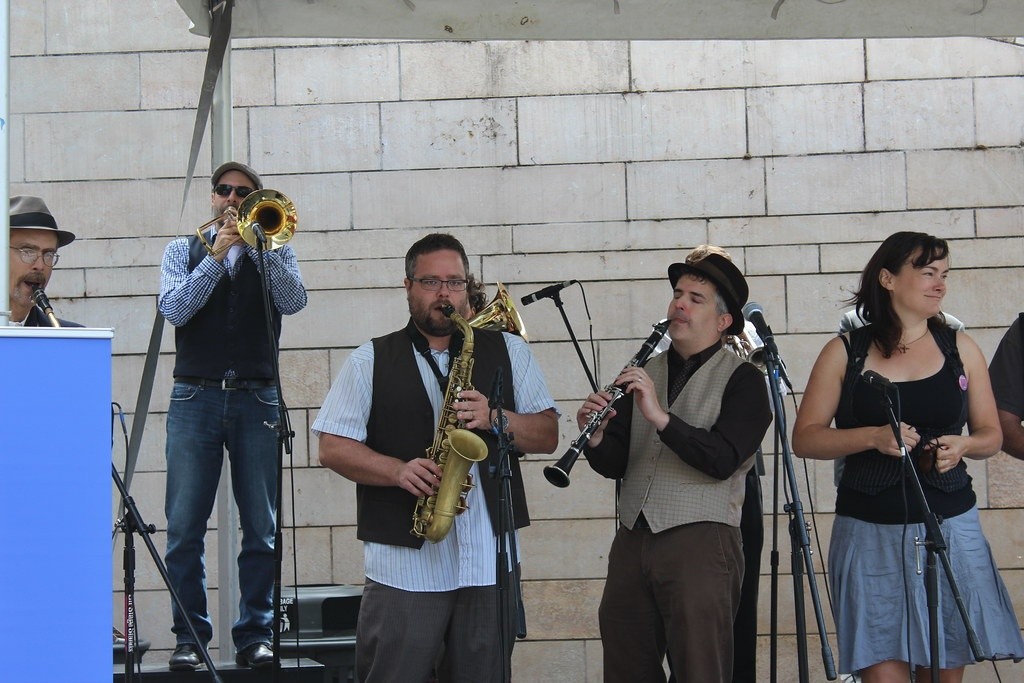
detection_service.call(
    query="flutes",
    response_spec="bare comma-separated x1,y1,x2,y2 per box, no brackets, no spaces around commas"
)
34,283,59,326
544,318,674,489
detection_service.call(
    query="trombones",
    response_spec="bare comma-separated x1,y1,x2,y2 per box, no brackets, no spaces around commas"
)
195,189,298,256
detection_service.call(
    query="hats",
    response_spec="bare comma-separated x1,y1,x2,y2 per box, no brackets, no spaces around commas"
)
9,195,75,248
211,160,264,190
668,253,749,337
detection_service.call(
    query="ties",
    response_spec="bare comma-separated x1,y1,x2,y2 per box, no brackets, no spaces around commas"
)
668,359,697,407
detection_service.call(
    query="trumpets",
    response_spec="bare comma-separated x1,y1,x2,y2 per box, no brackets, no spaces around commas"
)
468,282,531,344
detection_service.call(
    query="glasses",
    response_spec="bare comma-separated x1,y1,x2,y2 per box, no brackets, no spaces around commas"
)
9,246,61,268
408,278,470,292
212,184,253,197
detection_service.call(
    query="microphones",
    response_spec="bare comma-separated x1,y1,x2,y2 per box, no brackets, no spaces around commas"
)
488,368,503,410
251,220,267,243
520,280,577,307
742,301,778,355
863,369,897,391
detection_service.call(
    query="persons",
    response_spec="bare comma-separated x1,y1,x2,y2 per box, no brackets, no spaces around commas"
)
157,161,308,669
9,196,86,328
791,231,1024,683
577,244,773,683
311,233,563,683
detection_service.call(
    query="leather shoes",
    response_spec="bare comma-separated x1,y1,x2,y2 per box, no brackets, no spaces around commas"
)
169,644,206,670
235,643,281,668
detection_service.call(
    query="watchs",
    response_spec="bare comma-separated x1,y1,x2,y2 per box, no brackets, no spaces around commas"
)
487,409,509,433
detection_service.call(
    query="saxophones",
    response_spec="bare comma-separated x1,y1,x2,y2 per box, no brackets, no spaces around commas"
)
411,304,488,544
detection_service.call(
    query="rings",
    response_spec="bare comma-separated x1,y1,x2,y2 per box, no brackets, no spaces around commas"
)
639,377,642,383
